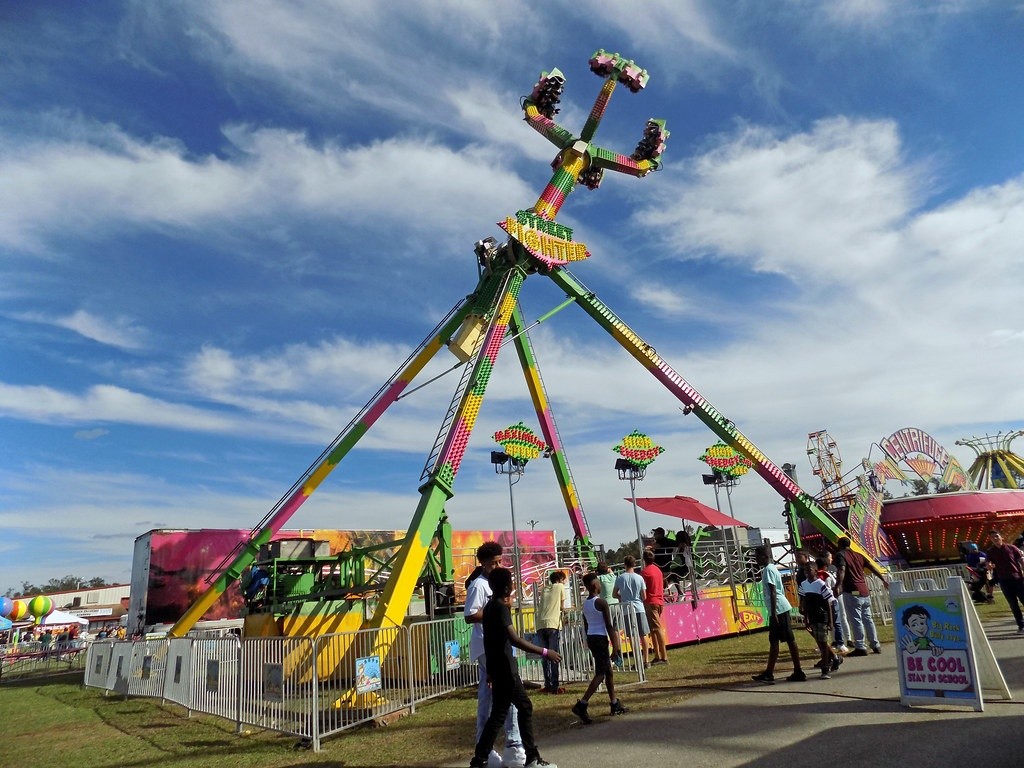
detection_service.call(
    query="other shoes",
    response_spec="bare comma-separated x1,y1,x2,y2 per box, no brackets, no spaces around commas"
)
553,688,566,695
847,640,855,648
820,666,831,679
1016,627,1023,634
539,687,552,693
830,642,838,650
838,643,848,652
643,662,652,669
830,656,844,671
631,663,645,671
847,648,868,657
987,594,994,599
676,593,685,603
814,658,832,668
650,658,669,666
873,647,881,653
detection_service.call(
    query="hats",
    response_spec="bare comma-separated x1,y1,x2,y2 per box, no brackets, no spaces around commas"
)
652,527,664,535
969,543,978,551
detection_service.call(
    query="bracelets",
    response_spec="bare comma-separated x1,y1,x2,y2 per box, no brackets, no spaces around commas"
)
540,647,548,658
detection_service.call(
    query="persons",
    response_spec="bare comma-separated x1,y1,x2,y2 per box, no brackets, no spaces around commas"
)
95,624,127,639
967,533,1024,632
749,546,807,683
571,573,629,721
539,571,566,694
647,527,693,602
596,549,670,671
0,624,79,660
795,535,891,679
461,541,561,768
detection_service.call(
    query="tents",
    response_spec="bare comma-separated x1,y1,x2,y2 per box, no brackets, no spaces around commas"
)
27,606,90,633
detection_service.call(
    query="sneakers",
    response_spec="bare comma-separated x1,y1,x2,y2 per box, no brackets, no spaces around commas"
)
786,671,806,681
609,698,629,714
751,672,775,684
572,698,593,724
503,746,526,768
486,750,503,768
524,758,557,768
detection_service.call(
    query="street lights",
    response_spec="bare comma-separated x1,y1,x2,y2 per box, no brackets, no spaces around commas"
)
702,474,738,601
614,459,649,570
714,470,746,581
490,451,529,639
